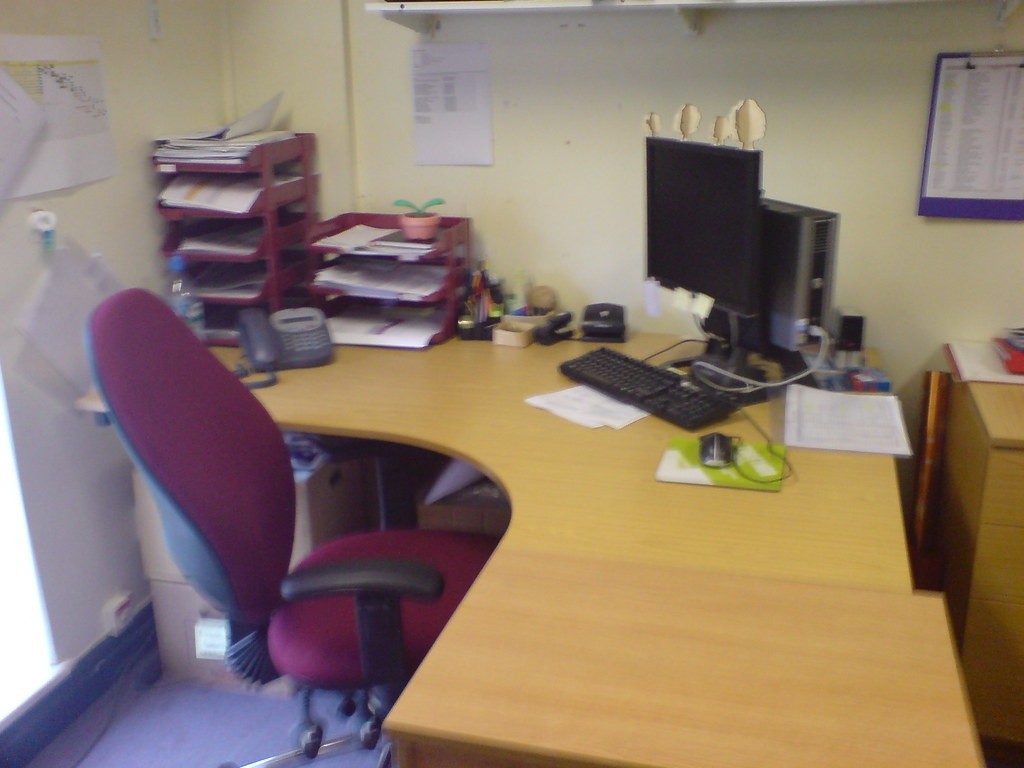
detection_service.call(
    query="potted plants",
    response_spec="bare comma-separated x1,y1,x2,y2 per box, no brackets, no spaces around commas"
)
391,197,448,242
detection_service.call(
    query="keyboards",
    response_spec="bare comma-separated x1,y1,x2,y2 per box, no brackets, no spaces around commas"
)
558,347,740,432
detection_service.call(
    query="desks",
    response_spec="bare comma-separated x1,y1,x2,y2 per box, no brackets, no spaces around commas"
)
927,341,1024,755
77,318,992,768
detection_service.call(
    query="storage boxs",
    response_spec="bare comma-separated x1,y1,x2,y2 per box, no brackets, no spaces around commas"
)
132,442,513,697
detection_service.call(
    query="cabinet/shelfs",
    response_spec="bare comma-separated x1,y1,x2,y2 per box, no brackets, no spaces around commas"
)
308,213,473,350
150,133,314,347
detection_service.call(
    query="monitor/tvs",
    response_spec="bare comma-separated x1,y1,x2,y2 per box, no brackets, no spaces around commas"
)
644,136,771,401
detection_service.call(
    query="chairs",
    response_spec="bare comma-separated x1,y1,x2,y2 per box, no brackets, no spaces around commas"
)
86,289,504,768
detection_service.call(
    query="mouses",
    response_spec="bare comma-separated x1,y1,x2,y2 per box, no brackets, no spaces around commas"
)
699,433,731,467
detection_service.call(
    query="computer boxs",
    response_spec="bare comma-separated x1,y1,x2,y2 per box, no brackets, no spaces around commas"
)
758,197,841,354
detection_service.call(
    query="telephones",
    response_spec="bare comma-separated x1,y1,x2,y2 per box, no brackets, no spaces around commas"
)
236,305,333,371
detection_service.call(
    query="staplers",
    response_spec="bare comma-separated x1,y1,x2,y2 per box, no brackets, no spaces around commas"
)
534,310,573,346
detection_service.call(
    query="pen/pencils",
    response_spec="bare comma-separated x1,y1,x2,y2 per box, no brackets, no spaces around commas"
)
466,269,489,324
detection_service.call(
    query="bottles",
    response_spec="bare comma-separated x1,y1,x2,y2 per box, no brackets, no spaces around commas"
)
160,256,203,346
